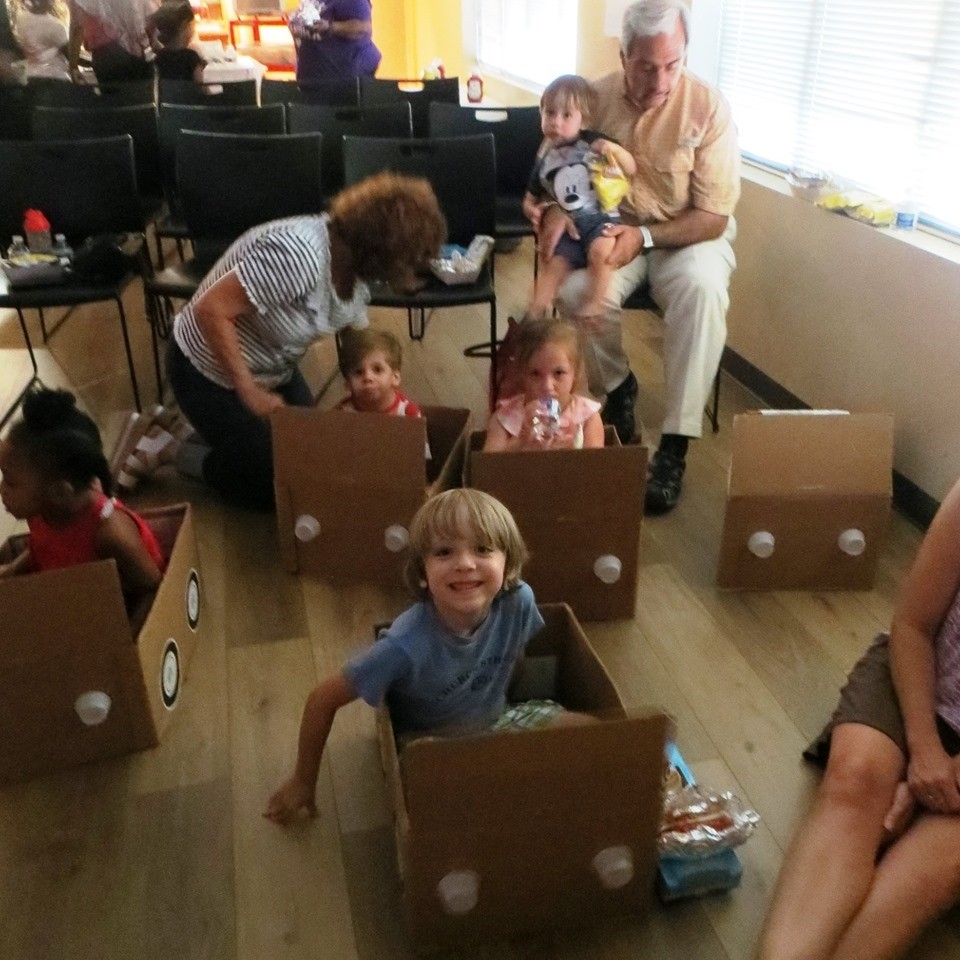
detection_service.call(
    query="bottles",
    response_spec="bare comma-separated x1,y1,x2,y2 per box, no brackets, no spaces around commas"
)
24,208,52,254
6,234,31,258
51,233,75,260
534,394,561,435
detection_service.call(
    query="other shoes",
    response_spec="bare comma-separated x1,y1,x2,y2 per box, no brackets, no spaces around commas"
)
108,412,172,497
644,452,687,513
599,371,639,444
146,402,189,442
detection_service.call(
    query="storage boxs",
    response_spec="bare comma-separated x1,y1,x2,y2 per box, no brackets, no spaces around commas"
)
464,422,648,622
716,407,896,592
371,603,670,957
0,502,205,778
266,397,472,588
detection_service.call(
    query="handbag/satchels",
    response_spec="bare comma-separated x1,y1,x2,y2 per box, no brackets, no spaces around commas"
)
69,232,176,338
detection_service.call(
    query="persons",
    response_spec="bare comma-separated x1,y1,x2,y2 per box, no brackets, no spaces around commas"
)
296,0,383,82
537,0,741,516
0,388,163,635
260,489,596,826
523,74,637,318
0,0,214,95
107,174,447,507
752,477,960,960
483,319,603,455
329,327,433,462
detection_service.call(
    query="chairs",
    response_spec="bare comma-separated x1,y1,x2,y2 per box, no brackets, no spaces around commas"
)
0,74,726,434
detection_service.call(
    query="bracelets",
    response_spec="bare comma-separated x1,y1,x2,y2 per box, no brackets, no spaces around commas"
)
327,19,333,36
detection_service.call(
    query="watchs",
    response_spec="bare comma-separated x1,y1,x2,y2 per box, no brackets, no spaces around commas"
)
639,225,654,256
